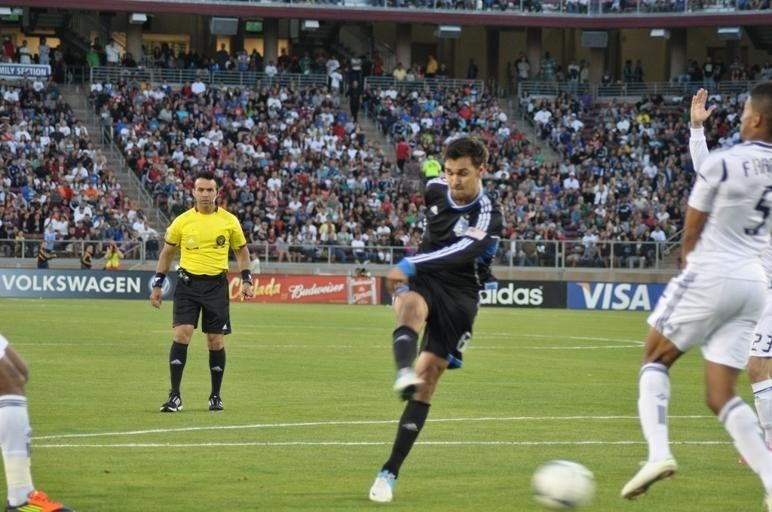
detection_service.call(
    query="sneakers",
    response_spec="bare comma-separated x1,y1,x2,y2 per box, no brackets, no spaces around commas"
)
160,393,183,412
620,457,677,500
209,395,224,410
4,492,74,512
369,471,396,502
393,374,425,400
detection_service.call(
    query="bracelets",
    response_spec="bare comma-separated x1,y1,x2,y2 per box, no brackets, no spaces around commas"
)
152,272,166,288
241,269,252,285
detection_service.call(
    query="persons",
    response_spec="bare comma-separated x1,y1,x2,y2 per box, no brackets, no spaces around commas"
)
0,333,76,512
620,80,772,510
1,1,772,270
149,168,257,414
366,137,505,503
688,88,772,453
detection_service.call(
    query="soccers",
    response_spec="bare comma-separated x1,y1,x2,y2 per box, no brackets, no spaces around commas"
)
532,460,594,509
